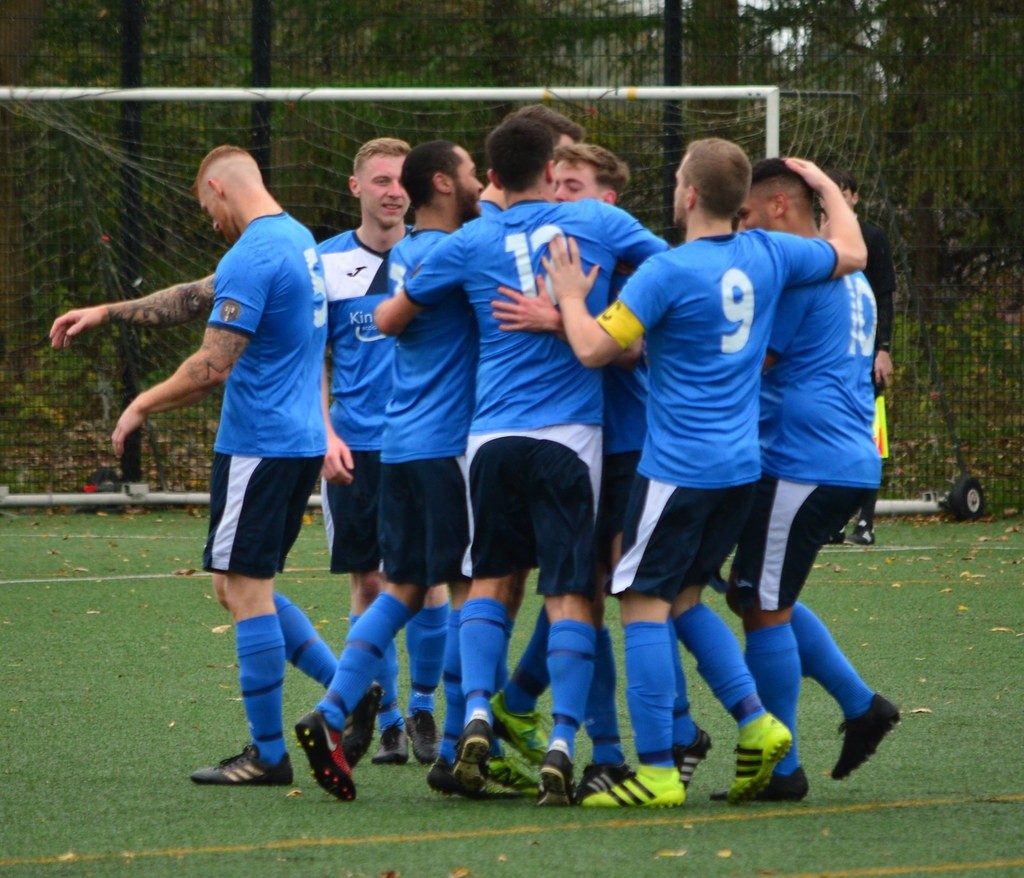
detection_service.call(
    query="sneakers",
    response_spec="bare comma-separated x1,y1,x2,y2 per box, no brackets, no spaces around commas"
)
190,743,294,786
710,764,809,801
572,763,634,805
452,719,493,798
373,717,410,762
428,756,485,800
582,765,686,809
672,722,712,788
404,710,440,762
727,713,793,804
291,710,358,801
342,680,384,769
829,694,901,779
537,751,574,806
485,754,544,796
488,692,553,764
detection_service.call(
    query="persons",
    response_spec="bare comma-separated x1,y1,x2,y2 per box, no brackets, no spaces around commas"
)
314,103,908,811
48,143,387,787
